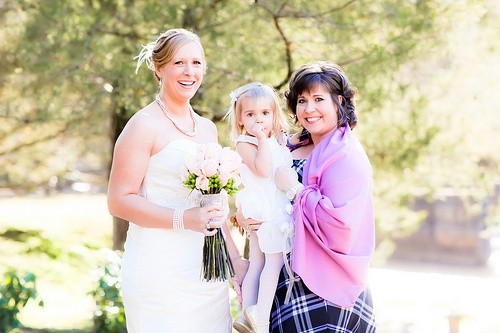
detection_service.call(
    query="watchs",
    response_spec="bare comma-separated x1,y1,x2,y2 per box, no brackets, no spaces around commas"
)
287,183,305,205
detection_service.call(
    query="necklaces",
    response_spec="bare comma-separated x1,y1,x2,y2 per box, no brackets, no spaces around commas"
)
156,93,200,137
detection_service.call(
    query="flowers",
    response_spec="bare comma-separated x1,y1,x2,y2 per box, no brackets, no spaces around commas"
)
180,142,247,282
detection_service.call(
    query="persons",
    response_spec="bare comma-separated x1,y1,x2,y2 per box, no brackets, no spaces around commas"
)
106,28,250,333
236,61,376,333
230,83,294,333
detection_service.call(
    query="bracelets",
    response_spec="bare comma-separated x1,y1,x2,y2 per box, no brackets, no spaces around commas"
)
232,256,243,259
173,209,185,231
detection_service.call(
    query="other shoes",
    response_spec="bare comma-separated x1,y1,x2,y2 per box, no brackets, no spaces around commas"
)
245,306,271,333
233,314,254,333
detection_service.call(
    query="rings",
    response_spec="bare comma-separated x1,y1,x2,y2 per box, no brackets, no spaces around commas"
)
247,225,251,231
207,223,210,230
